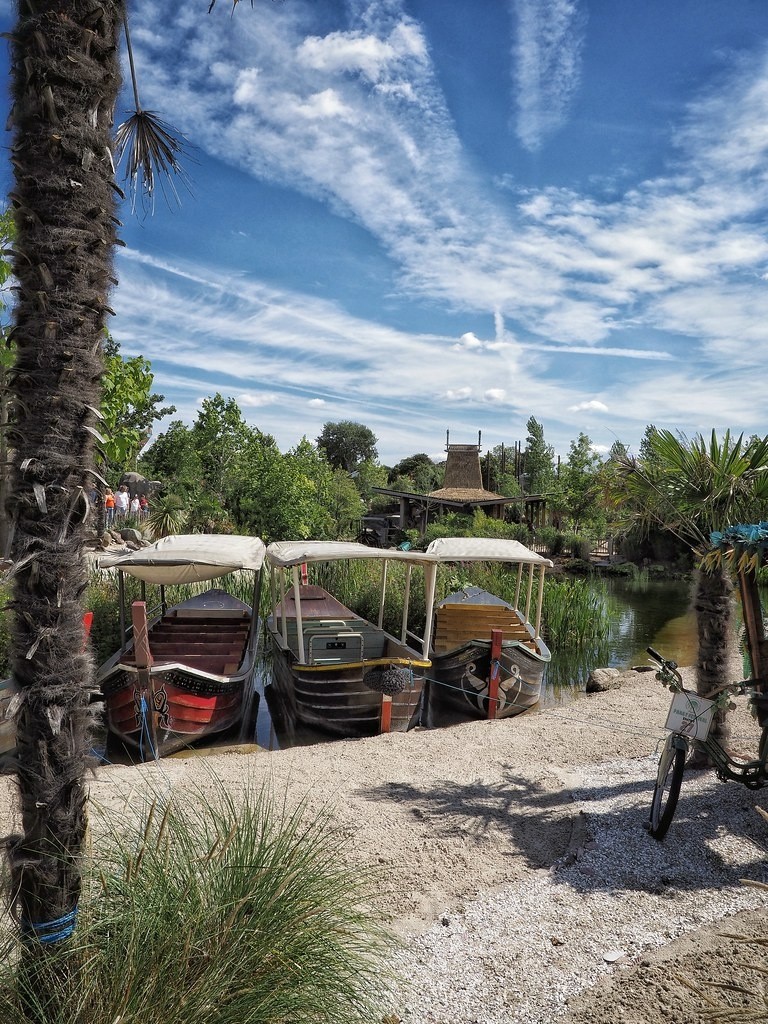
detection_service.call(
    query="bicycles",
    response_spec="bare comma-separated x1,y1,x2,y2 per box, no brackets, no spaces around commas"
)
632,645,768,838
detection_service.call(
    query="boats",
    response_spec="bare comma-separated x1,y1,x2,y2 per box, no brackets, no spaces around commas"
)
263,539,441,737
422,537,553,720
86,535,267,759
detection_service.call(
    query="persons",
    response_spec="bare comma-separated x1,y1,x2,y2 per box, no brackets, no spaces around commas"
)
92,484,150,529
526,520,535,530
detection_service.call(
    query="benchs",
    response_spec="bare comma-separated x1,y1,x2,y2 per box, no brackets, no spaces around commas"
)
121,624,249,671
279,619,386,661
435,603,535,652
